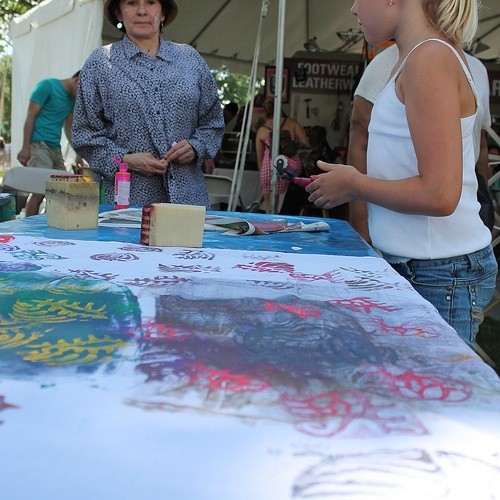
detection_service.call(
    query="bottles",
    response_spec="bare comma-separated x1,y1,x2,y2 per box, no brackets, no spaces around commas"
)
113,156,131,210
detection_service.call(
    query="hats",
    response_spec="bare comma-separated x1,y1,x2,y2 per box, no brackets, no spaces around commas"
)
103,0,178,33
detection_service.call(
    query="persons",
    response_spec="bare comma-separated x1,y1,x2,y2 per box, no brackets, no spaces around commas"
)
70,0,225,210
16,71,81,216
202,0,500,352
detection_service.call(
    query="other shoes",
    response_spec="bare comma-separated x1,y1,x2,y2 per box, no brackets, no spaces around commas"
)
246,202,260,213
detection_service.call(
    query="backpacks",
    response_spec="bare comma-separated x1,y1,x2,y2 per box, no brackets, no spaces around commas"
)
261,115,297,159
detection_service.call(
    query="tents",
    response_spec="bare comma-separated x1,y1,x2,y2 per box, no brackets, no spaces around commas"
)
9,0,500,232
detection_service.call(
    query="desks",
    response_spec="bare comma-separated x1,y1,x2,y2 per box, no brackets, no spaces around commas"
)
0,204,500,499
